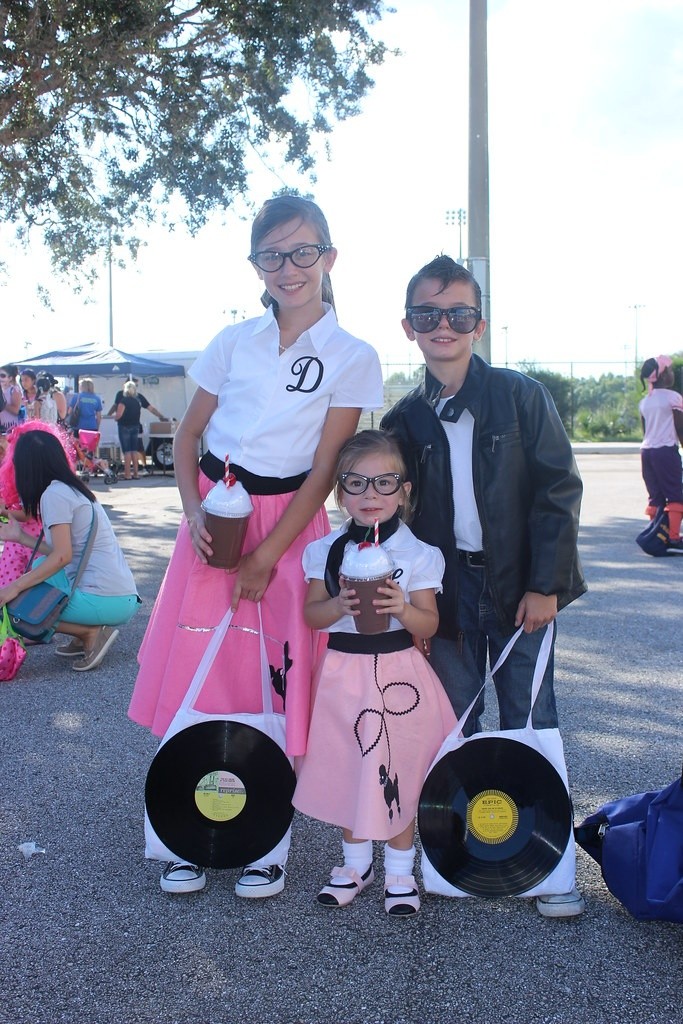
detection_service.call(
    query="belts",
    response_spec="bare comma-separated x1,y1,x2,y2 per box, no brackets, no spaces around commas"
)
455,549,486,568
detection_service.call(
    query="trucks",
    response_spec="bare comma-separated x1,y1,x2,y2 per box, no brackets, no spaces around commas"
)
62,352,202,471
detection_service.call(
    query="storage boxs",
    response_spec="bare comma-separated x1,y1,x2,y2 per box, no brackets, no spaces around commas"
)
150,421,181,433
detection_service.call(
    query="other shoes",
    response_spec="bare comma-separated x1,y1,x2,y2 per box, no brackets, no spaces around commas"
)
122,467,150,479
73,624,120,671
56,642,85,656
667,537,683,552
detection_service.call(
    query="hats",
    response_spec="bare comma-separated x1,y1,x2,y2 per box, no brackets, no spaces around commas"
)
43,373,59,384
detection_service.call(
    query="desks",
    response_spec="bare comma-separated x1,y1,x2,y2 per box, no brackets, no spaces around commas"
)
97,419,121,465
137,434,175,478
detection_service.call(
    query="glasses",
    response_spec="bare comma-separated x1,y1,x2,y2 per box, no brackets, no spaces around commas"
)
336,472,407,495
0,373,10,378
247,244,331,273
406,306,482,335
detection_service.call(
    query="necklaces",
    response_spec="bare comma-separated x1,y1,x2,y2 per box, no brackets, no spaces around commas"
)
280,346,287,350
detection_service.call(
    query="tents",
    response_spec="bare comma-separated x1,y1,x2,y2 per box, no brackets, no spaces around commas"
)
0,341,188,410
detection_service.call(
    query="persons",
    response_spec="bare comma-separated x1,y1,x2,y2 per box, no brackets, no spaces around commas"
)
126,198,384,898
70,380,102,435
115,381,140,480
301,429,458,915
380,255,586,917
0,419,74,644
640,355,683,547
107,377,168,476
0,430,142,670
0,364,67,450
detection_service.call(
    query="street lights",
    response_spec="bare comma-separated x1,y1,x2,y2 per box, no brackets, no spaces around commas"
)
502,327,507,368
445,208,466,266
628,304,646,368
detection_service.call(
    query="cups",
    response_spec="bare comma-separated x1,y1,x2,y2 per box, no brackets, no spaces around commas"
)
338,541,394,635
200,473,255,569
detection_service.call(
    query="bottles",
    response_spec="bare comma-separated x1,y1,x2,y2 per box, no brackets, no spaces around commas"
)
18,405,27,421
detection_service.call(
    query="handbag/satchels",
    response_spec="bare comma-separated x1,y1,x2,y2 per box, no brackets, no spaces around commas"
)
575,776,683,924
418,620,576,898
1,574,69,641
65,404,78,425
0,606,32,680
636,513,670,555
144,601,297,869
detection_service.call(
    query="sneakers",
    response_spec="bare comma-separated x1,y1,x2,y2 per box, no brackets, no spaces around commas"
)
235,864,288,897
536,888,585,917
159,861,206,893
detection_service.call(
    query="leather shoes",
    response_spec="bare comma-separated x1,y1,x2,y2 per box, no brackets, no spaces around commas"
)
316,867,375,907
382,877,421,917
